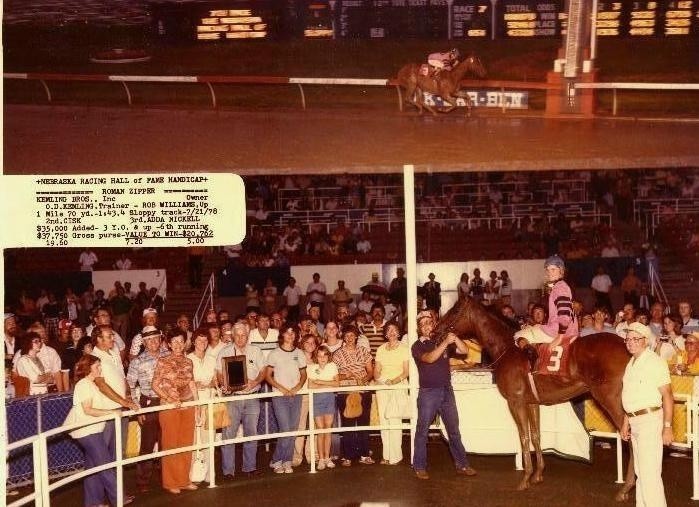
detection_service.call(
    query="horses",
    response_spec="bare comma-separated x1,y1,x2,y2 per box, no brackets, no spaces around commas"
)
396,53,487,118
429,285,636,500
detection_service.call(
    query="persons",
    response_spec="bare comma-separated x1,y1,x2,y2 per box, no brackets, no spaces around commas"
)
407,305,477,478
150,326,204,494
428,45,459,71
5,170,696,497
510,253,582,361
63,355,134,506
619,321,678,507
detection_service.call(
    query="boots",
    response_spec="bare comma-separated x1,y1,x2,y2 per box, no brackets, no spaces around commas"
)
524,345,540,372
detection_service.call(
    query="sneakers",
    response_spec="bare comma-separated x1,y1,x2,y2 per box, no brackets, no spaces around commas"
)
416,469,430,480
285,465,293,474
457,466,476,475
317,458,326,470
326,457,336,468
274,466,285,475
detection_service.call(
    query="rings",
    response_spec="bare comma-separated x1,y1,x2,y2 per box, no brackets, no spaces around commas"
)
177,404,180,406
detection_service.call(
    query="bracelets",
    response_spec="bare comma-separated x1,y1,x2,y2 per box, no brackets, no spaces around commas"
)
164,395,169,401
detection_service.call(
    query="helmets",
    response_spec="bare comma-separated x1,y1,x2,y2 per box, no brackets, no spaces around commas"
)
544,257,566,269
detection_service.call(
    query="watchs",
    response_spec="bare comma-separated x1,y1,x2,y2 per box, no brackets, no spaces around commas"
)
662,421,672,428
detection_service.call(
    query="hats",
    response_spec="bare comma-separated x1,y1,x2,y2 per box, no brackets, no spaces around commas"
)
141,326,162,339
143,308,158,316
622,323,652,338
415,310,437,322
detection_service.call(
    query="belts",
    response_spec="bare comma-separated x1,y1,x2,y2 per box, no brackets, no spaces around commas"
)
627,407,662,417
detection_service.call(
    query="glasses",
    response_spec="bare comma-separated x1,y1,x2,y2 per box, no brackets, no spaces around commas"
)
418,321,434,327
624,336,645,343
